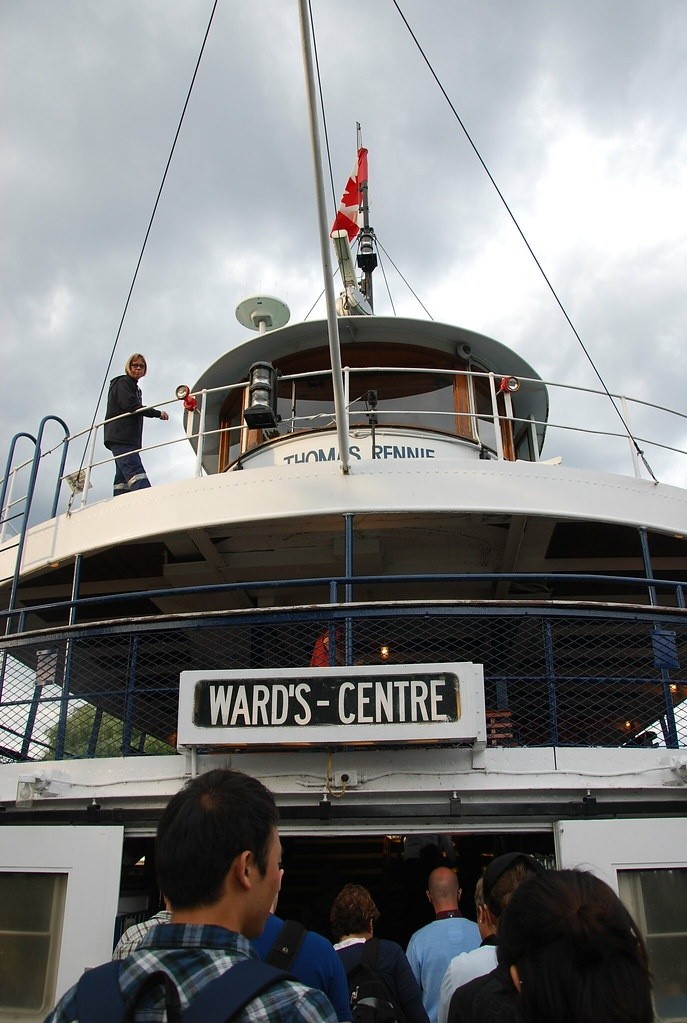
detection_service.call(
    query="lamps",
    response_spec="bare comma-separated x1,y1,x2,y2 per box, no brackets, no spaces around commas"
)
381,641,389,659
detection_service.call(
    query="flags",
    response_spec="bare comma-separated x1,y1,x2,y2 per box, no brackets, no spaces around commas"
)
330,162,363,243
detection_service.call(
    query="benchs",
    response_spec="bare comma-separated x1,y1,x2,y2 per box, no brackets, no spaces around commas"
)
487,709,519,747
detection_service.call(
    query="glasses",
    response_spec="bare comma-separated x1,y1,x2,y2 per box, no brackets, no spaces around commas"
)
131,363,145,368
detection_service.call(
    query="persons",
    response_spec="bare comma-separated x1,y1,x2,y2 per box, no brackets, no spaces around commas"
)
406,867,482,1023
248,868,352,1023
50,769,339,1023
104,354,169,496
499,870,654,1023
112,893,172,960
439,879,498,1023
446,858,547,1023
332,884,430,1023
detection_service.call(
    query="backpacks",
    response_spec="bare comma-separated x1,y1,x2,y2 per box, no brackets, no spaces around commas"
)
345,937,407,1023
